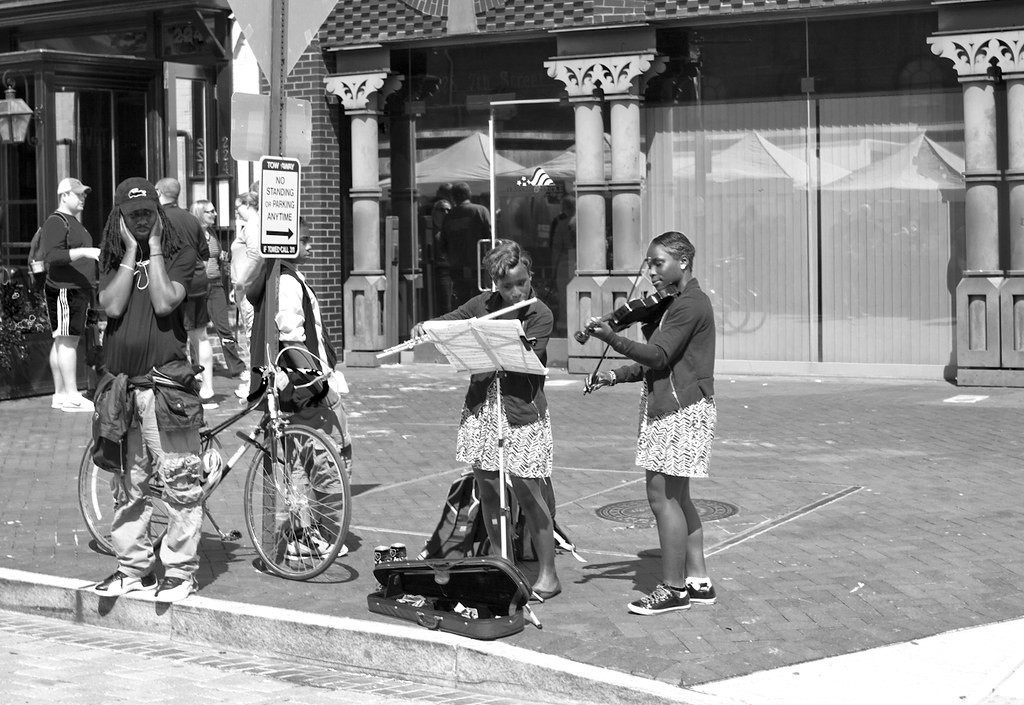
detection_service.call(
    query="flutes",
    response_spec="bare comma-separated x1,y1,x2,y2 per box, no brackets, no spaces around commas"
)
375,296,538,360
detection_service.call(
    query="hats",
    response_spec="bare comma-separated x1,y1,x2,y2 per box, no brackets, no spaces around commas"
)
115,177,159,215
56,177,92,194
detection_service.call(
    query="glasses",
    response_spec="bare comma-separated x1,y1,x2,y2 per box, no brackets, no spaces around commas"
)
235,203,243,211
203,209,214,213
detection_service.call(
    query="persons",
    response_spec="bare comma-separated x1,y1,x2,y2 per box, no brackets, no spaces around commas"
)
40,178,101,413
96,177,206,602
154,177,259,409
431,181,574,338
409,241,563,601
589,231,719,615
246,220,350,562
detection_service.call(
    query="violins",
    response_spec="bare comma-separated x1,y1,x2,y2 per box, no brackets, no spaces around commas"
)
573,283,683,345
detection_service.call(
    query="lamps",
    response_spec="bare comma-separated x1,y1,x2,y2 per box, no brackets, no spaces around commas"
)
0,86,43,145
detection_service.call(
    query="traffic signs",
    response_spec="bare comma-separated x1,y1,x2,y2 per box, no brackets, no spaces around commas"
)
259,155,301,260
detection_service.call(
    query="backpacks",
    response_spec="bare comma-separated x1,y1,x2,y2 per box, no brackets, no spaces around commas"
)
419,474,518,563
27,211,70,293
515,477,577,562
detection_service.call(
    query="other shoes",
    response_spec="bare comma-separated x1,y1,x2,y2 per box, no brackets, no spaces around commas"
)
528,578,561,601
51,394,95,412
154,577,198,602
200,394,219,410
93,570,144,597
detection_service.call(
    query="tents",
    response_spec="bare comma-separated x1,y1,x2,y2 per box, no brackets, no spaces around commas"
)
378,132,964,202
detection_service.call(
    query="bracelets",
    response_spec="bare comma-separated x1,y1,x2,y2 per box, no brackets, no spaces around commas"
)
150,254,164,257
120,264,134,271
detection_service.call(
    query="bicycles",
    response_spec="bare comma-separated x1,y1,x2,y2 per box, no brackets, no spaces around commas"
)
76,343,352,582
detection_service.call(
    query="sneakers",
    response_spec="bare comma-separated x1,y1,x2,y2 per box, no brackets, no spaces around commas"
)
282,527,349,561
686,581,718,607
627,583,692,615
